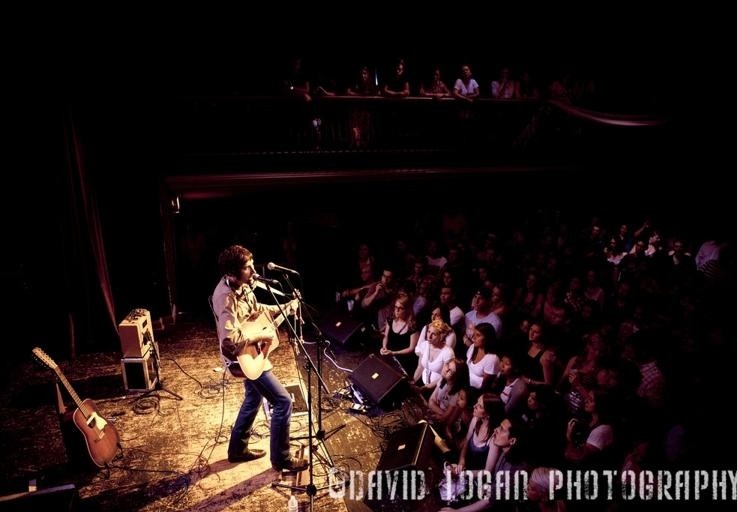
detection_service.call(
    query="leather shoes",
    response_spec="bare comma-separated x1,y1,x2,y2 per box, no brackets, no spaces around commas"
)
228,450,265,461
271,455,307,471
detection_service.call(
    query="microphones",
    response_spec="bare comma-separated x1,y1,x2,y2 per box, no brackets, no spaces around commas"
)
268,261,299,275
252,273,279,285
144,332,152,341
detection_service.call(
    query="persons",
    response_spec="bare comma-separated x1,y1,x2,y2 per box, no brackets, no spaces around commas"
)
310,59,343,152
512,66,538,153
488,64,516,100
282,54,323,153
451,62,481,123
511,76,595,153
382,55,410,100
344,61,384,150
208,241,308,472
416,67,453,99
336,189,735,509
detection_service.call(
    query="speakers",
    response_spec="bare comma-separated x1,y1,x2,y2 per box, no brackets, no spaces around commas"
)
374,421,435,486
119,309,155,357
327,317,373,353
348,354,411,410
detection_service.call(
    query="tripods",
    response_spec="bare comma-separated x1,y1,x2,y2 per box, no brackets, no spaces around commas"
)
265,283,351,512
135,340,183,403
282,273,346,466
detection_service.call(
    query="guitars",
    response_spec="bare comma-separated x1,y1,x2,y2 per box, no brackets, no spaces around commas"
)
222,290,302,380
32,347,120,468
